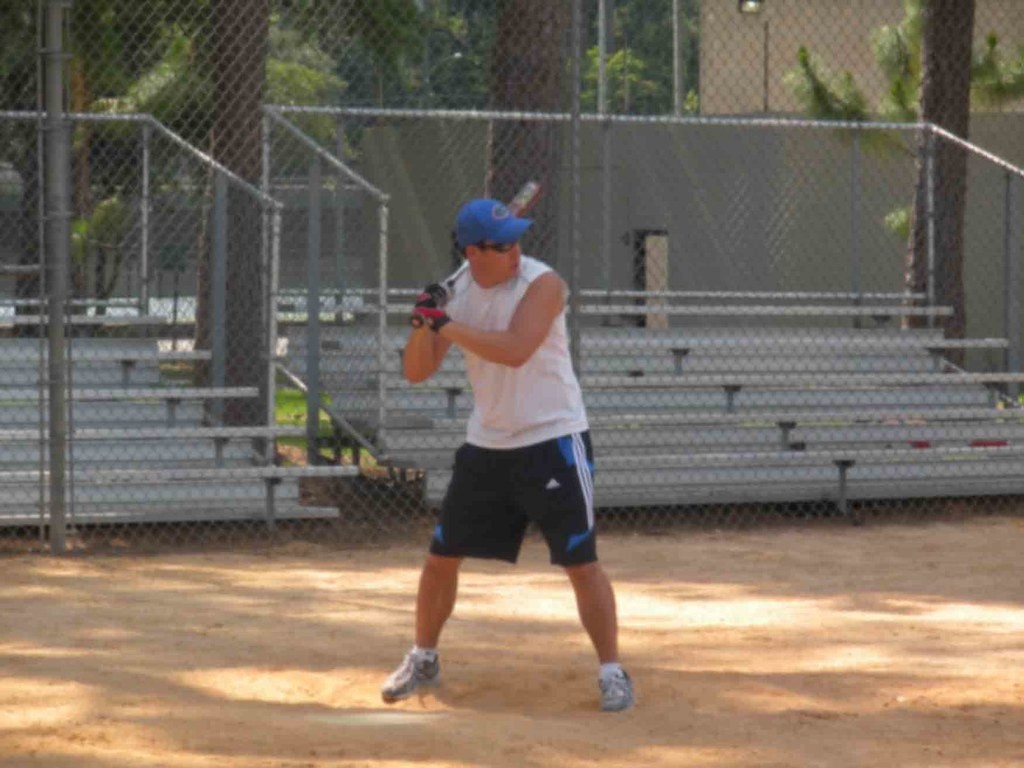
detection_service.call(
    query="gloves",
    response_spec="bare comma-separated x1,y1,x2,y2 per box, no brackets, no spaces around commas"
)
414,282,452,309
412,306,449,333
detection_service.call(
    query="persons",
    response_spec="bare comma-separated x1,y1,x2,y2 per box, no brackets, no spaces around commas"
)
381,199,635,711
89,173,132,316
1,25,79,336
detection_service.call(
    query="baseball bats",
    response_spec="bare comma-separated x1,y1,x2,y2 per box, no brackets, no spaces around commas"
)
408,179,542,329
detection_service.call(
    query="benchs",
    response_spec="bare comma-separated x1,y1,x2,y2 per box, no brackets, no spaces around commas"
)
0,307,1024,529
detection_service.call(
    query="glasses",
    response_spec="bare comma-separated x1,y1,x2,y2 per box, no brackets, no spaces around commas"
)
483,240,517,252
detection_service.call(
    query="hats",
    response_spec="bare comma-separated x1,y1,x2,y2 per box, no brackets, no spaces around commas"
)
453,199,534,249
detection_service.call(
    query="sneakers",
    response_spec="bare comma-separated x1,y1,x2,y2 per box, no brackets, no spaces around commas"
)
381,650,440,699
598,669,630,711
